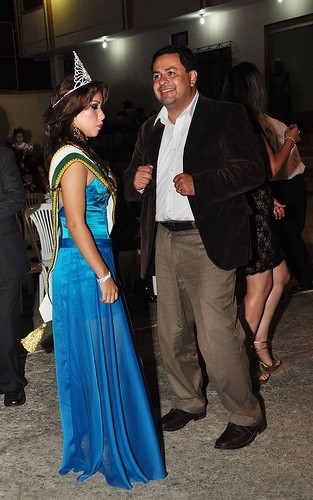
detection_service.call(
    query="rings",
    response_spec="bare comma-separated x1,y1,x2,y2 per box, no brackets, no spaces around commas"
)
297,130,300,133
273,211,276,212
177,187,181,191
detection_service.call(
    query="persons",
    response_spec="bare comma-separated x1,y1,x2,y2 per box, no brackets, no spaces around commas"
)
263,114,313,295
0,145,29,406
11,127,34,159
221,61,301,383
120,46,268,449
40,50,168,490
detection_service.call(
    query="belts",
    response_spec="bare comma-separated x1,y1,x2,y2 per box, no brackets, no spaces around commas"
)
161,219,196,233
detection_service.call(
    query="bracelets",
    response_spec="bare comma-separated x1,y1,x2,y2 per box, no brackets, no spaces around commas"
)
96,271,112,284
286,136,295,145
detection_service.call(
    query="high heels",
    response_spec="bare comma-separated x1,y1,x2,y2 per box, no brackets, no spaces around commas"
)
252,342,279,372
248,367,268,385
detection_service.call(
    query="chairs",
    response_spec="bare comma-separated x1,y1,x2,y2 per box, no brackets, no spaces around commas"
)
15,192,52,315
24,203,54,270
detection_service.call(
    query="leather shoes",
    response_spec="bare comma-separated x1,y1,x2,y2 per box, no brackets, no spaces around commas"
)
4,389,26,407
215,420,266,451
158,408,206,431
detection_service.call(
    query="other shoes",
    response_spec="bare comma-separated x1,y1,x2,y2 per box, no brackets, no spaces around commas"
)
291,282,313,294
